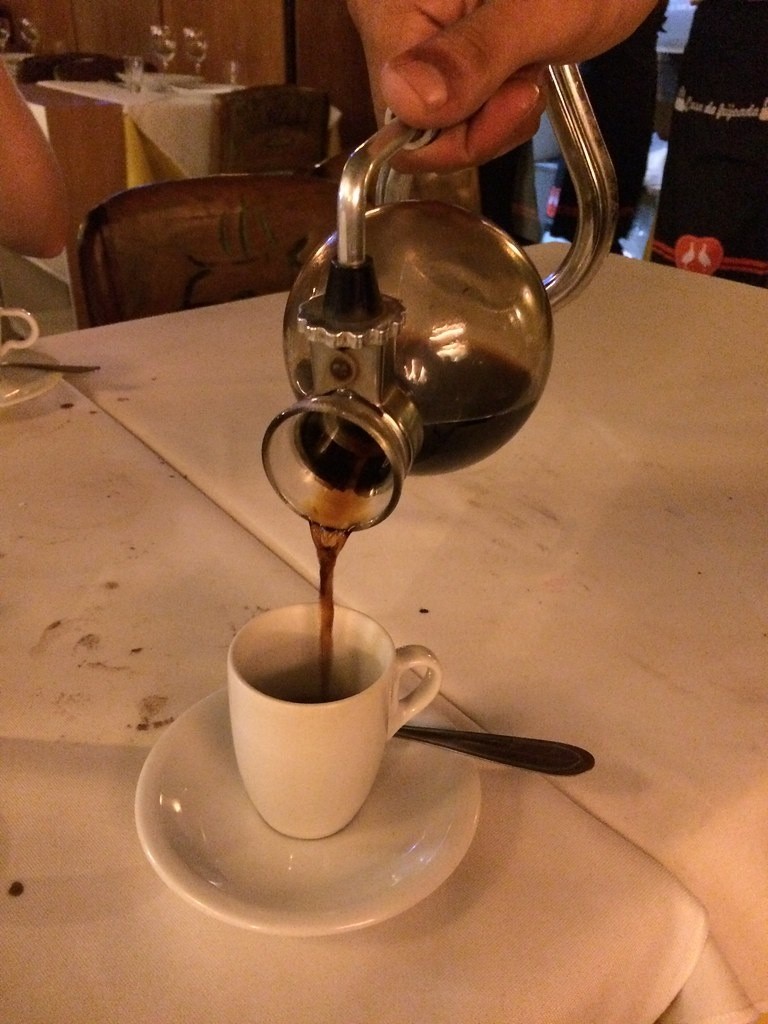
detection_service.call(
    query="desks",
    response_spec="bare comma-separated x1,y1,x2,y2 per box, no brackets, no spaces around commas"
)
2,50,343,284
0,240,768,1024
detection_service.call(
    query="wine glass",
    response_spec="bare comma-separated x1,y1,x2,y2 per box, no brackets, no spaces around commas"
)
0,18,10,57
185,28,209,86
20,21,40,57
150,24,176,91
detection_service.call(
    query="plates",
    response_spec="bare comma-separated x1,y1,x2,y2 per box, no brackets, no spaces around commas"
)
135,686,481,936
0,348,62,408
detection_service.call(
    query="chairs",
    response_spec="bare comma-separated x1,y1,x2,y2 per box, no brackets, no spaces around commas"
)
209,82,329,177
56,172,380,333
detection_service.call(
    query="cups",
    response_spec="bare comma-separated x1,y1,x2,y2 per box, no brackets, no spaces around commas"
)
228,603,442,840
225,61,239,83
123,56,143,81
0,306,40,359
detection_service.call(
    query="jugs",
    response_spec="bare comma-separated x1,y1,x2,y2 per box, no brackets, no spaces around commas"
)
261,118,555,532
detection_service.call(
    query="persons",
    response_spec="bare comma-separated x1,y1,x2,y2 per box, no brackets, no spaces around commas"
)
0,61,69,258
350,1,767,286
